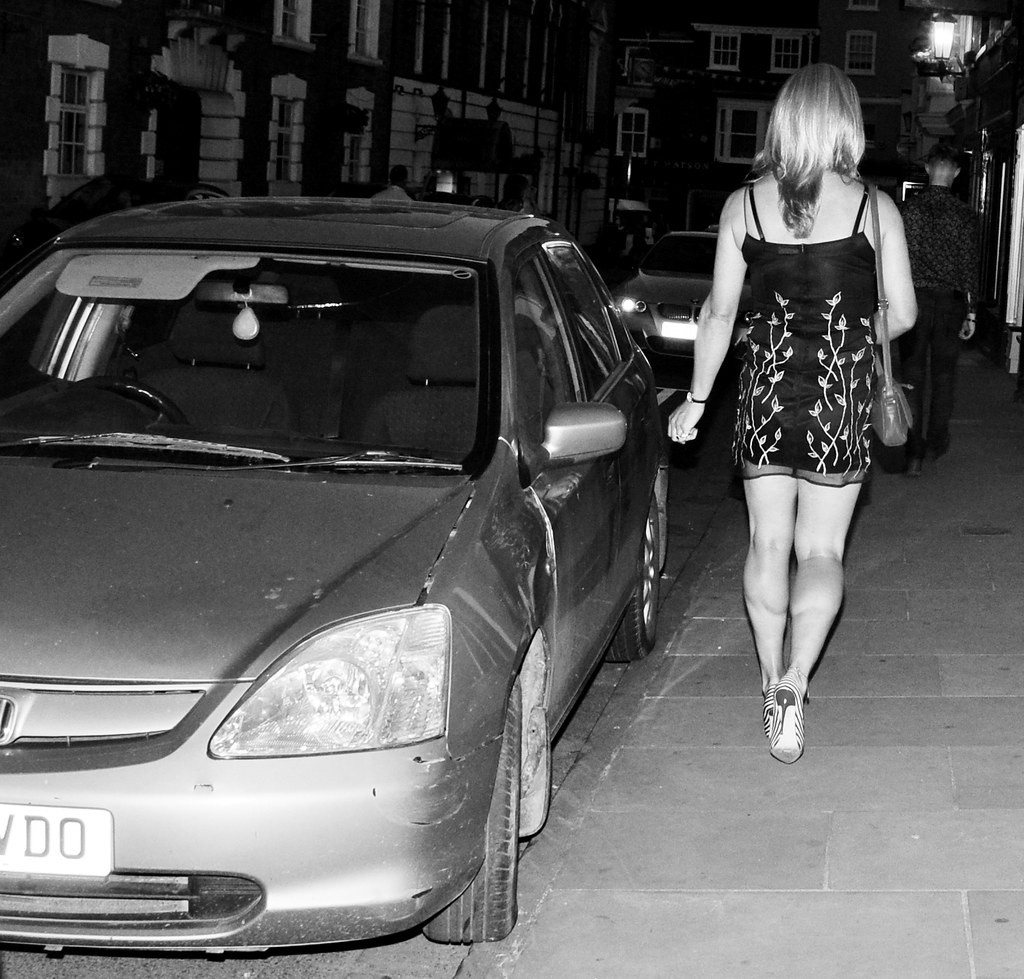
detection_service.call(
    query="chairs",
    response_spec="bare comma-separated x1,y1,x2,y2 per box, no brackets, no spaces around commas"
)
134,304,292,431
363,303,478,459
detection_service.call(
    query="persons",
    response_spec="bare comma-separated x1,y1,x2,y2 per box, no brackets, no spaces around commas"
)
901,140,983,479
492,174,543,217
661,64,919,767
367,165,415,204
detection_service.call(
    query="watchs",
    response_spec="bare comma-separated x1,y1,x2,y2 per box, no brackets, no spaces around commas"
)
687,391,707,404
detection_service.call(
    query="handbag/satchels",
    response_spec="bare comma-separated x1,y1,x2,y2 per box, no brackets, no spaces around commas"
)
869,351,913,447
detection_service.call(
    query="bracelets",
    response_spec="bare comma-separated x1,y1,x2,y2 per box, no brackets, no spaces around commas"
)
964,318,976,322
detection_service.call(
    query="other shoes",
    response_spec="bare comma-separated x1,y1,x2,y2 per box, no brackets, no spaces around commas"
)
906,432,951,474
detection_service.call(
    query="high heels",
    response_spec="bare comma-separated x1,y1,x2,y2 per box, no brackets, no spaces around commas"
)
764,680,804,764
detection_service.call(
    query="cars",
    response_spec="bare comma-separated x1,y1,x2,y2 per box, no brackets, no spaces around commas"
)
606,231,753,372
0,194,676,958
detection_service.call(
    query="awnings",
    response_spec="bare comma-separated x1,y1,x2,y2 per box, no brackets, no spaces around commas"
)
430,115,514,174
